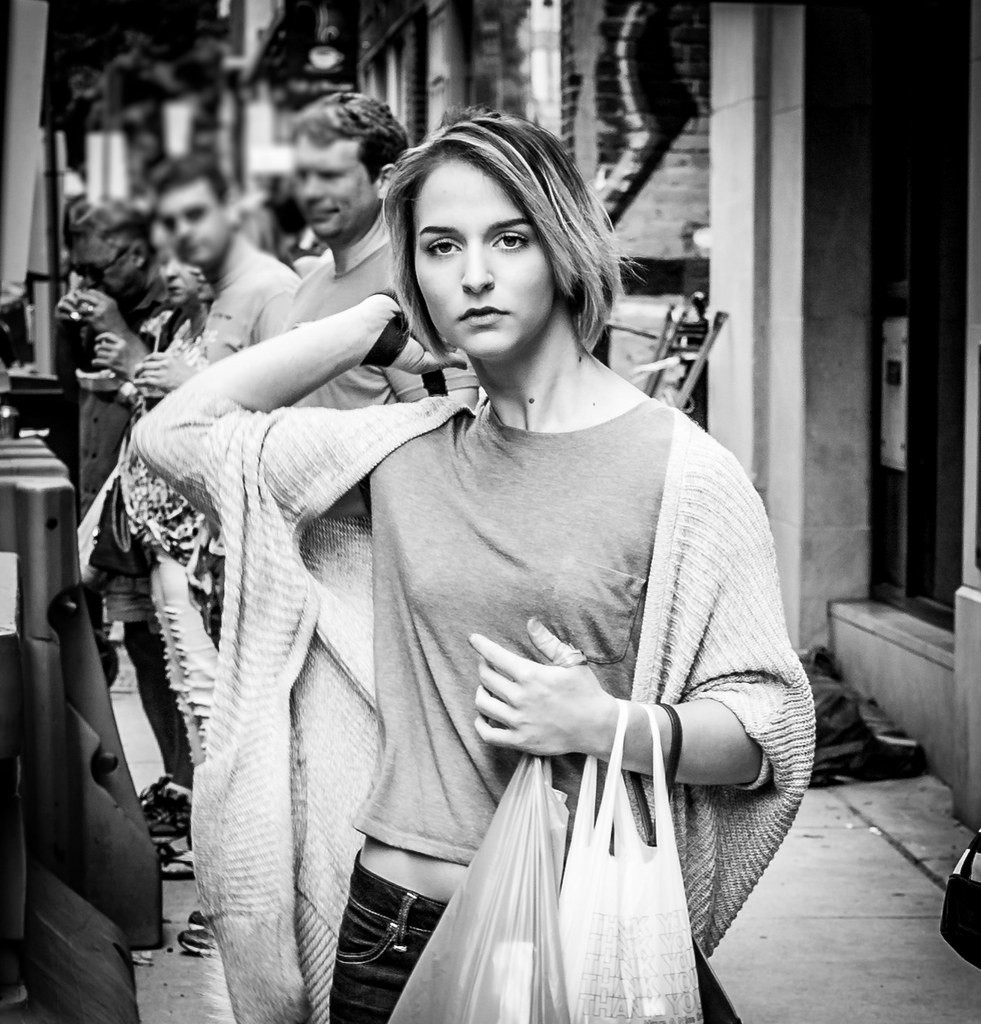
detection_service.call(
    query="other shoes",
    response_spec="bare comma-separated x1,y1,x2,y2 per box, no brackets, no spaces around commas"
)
159,849,194,879
139,775,192,842
176,930,221,957
188,912,210,930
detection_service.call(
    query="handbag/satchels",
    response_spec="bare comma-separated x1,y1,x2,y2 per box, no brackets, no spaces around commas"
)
388,698,704,1024
87,476,160,576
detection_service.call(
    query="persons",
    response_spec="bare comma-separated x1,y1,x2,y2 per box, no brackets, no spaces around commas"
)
247,90,479,415
56,158,296,956
130,108,818,1024
